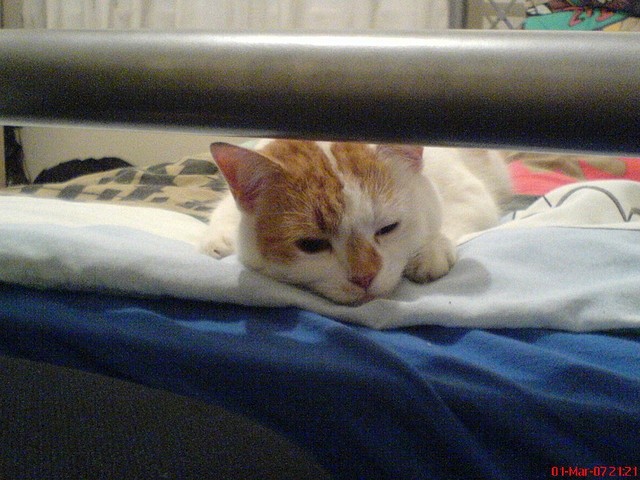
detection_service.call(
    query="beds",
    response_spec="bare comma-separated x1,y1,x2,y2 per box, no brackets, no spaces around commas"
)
0,1,640,480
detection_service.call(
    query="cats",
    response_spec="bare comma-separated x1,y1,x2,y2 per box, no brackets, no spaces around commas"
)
200,137,515,307
